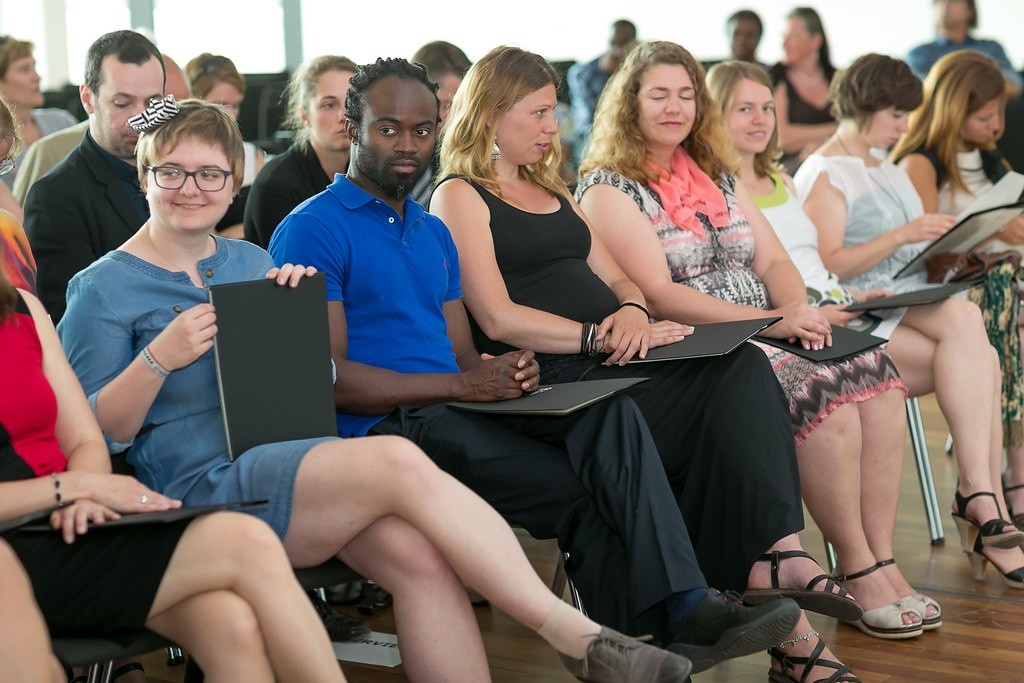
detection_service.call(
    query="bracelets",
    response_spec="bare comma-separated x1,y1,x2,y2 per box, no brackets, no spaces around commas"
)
140,346,170,379
53,472,62,506
619,302,650,320
581,322,607,356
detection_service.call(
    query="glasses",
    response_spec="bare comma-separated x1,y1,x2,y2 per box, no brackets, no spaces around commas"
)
145,166,234,192
0,154,16,176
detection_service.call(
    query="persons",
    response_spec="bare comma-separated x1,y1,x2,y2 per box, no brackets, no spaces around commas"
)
428,42,1024,683
54,57,802,683
0,30,272,329
0,538,67,683
554,0,1014,173
243,42,473,252
0,214,351,683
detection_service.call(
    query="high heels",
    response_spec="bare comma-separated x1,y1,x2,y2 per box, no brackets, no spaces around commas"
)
875,558,942,631
830,561,923,639
963,527,1024,589
951,491,1024,552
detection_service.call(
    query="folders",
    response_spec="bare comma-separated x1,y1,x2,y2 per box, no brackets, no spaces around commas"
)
13,498,268,534
893,192,1024,282
749,323,889,361
599,316,783,365
208,274,338,463
839,279,985,311
447,376,652,416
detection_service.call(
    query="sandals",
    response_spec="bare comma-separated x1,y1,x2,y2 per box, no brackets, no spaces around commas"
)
743,548,862,622
765,637,863,683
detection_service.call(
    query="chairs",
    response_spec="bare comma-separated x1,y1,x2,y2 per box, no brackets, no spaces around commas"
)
55,630,185,683
294,557,364,591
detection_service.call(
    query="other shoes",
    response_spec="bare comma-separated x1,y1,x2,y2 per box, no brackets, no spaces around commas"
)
558,624,691,683
639,587,802,675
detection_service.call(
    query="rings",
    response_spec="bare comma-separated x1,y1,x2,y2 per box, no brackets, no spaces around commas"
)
141,494,147,503
